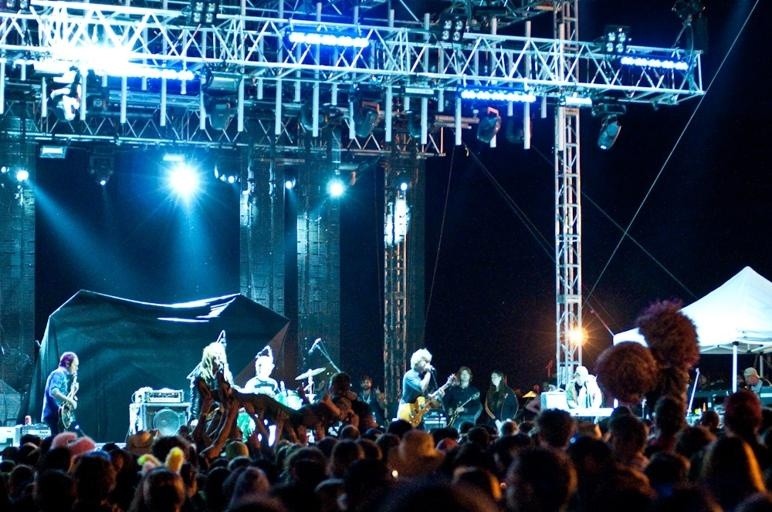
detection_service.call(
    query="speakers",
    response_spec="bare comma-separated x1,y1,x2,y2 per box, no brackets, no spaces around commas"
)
128,403,190,437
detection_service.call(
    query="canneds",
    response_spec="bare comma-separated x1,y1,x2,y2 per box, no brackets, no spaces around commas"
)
24,415,32,425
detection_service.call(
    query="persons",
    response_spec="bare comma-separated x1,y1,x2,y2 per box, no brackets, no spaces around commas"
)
0,343,772,512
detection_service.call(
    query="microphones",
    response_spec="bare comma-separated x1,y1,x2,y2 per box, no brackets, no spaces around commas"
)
307,338,322,357
219,338,227,348
431,368,435,371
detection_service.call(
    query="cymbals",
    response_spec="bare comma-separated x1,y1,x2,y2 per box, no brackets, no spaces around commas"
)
295,367,326,380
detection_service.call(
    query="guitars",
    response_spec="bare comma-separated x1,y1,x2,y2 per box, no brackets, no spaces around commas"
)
61,370,77,429
398,373,457,427
447,392,480,428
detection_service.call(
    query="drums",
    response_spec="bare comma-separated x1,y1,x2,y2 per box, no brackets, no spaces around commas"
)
274,389,302,411
304,393,318,405
